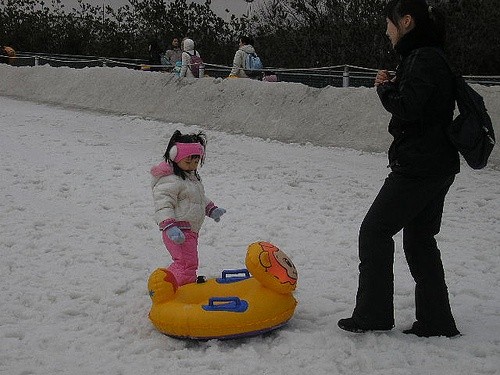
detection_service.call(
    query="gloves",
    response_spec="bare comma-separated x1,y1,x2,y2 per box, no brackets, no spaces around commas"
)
209,207,226,223
166,226,186,245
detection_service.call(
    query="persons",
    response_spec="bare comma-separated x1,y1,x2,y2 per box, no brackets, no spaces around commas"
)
163,37,182,64
180,37,203,79
262,72,277,83
150,129,226,286
336,0,461,340
229,36,259,80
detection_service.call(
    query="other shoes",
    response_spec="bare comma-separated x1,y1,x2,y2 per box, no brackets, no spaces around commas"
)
402,323,461,341
337,317,396,335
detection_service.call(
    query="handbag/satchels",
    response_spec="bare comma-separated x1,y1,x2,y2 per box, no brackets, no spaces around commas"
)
401,45,496,171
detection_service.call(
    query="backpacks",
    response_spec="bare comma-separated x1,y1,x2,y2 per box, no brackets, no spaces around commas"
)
238,49,264,77
183,49,205,78
